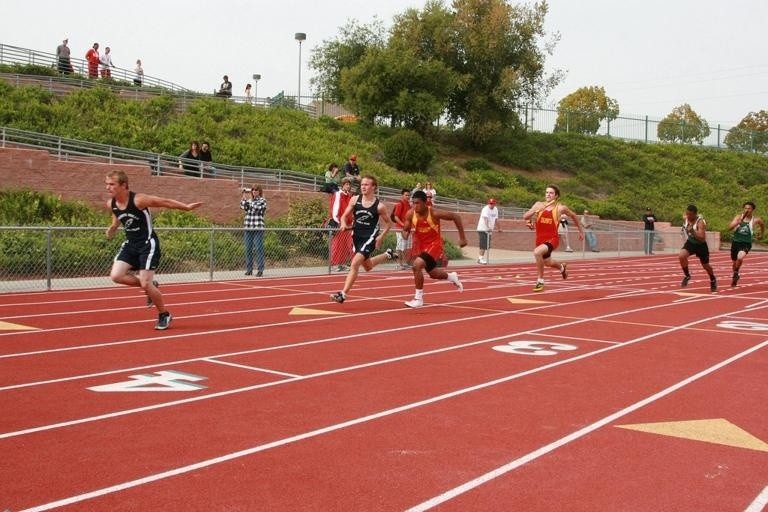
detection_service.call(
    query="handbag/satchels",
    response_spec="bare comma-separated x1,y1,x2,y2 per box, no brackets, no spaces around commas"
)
321,219,339,235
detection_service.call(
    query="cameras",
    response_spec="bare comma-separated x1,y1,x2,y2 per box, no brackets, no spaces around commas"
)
242,188,251,192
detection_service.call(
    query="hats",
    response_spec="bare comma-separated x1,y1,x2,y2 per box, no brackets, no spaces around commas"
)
350,155,356,160
488,199,497,204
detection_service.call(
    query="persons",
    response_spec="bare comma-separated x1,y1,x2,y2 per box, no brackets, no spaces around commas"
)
475,198,502,266
179,140,201,178
522,184,586,292
728,201,766,288
425,192,433,206
330,179,355,273
325,163,341,193
411,182,422,204
422,182,437,204
557,214,574,253
103,168,206,333
394,189,414,271
148,147,164,176
344,154,362,182
580,208,601,253
677,205,718,293
328,175,400,304
55,38,75,78
643,206,657,256
245,84,254,105
84,42,105,79
197,142,216,178
400,191,469,309
239,183,269,279
218,75,233,99
99,47,116,80
132,59,145,87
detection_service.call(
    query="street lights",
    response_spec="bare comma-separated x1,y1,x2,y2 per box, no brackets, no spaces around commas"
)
252,73,262,107
293,33,307,108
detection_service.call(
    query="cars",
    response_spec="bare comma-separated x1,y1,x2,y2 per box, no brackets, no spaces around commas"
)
336,114,361,122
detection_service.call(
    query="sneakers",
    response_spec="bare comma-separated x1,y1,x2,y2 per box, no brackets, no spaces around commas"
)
560,263,568,279
155,311,172,330
531,282,544,291
331,291,347,304
710,277,717,291
681,276,691,287
452,272,463,293
146,280,159,308
731,273,739,286
404,298,423,307
385,249,399,260
